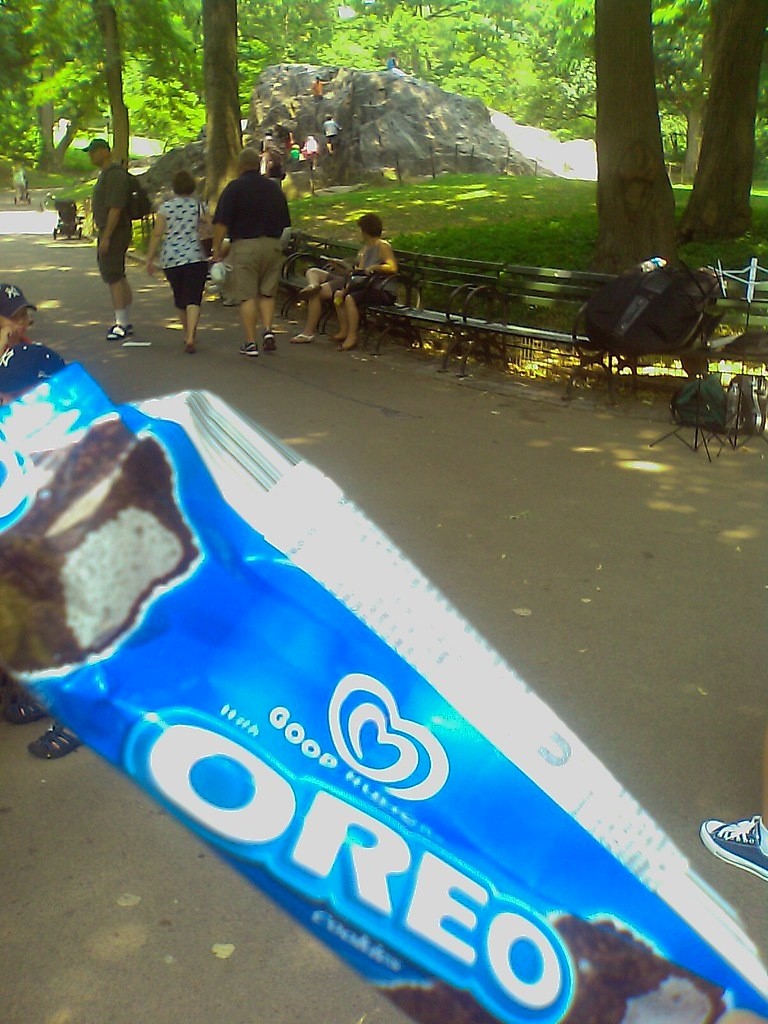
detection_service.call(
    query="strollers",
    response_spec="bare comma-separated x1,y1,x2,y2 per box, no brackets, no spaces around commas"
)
53,200,84,240
14,176,31,205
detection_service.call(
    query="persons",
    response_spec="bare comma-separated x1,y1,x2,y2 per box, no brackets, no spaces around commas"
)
146,170,211,353
388,51,413,75
81,139,134,340
309,76,330,101
293,213,399,351
212,147,292,357
700,722,768,882
11,156,26,204
205,226,292,306
0,282,90,759
260,114,343,185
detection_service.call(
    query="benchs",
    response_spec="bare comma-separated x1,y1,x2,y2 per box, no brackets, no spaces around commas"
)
276,231,768,434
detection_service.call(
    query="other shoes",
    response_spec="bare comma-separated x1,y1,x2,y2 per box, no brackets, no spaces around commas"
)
263,327,276,351
183,339,196,353
330,332,359,350
239,342,260,356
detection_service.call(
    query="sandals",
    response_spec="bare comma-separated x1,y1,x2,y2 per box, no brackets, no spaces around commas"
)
5,701,47,725
106,325,134,340
28,722,82,760
289,334,315,343
299,284,322,296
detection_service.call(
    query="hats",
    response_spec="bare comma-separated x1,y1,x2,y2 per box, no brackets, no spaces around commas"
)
82,138,111,152
0,284,38,317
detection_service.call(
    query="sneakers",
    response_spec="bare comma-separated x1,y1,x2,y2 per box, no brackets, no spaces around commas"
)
699,815,768,882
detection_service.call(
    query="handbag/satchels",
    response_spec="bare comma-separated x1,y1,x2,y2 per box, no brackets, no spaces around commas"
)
669,373,727,433
350,273,372,289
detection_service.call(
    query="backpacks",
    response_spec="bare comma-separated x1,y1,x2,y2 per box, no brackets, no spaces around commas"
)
586,257,724,357
106,165,152,219
727,374,767,434
